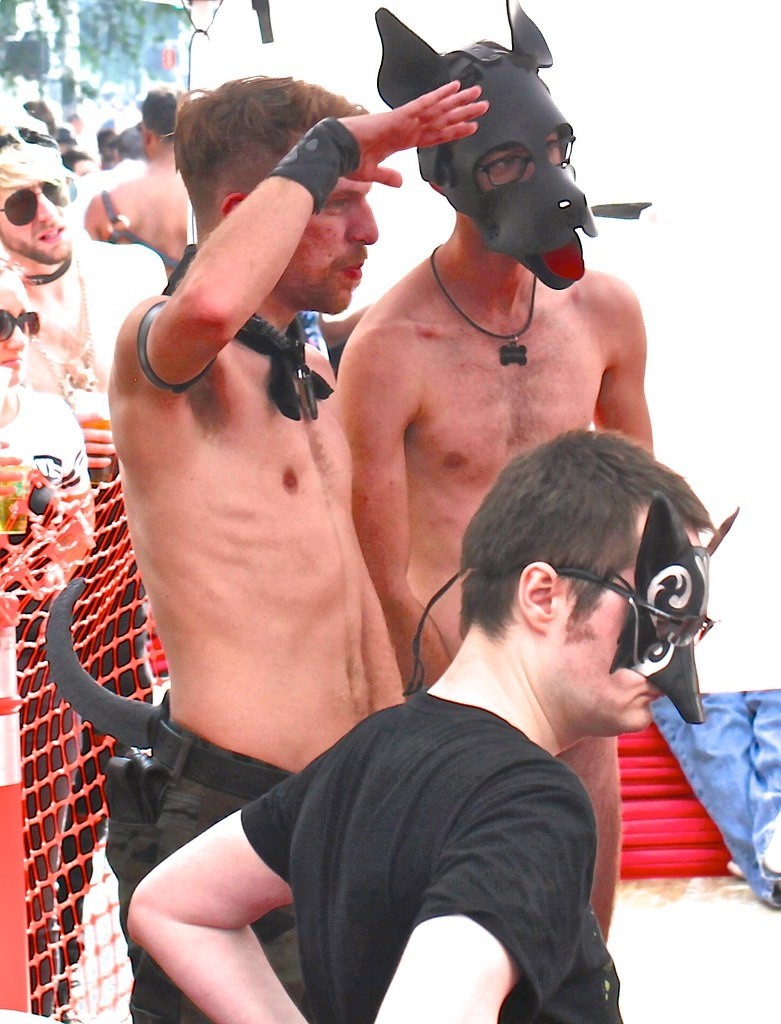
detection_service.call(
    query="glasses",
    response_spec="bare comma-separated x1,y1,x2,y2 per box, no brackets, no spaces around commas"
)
0,176,78,226
555,567,716,648
476,135,576,187
0,309,41,342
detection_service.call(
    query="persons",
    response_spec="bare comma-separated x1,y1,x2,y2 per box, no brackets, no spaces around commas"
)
1,1,650,1024
127,423,741,1024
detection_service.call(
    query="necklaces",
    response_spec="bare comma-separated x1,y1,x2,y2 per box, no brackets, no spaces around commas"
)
429,248,540,368
20,256,72,283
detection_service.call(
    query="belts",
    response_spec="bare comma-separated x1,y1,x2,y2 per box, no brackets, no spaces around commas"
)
151,718,295,801
